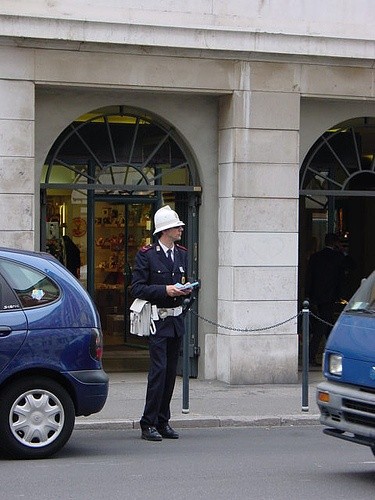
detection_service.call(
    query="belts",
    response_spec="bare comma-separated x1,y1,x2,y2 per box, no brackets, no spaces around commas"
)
159,306,183,316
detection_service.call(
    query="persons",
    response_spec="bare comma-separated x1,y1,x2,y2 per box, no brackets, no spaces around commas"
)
305,233,356,339
130,205,193,441
49,223,80,279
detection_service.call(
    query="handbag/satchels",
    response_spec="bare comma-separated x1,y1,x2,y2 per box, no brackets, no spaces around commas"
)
130,298,151,337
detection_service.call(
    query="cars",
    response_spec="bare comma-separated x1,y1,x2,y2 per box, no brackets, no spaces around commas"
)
0,246,111,460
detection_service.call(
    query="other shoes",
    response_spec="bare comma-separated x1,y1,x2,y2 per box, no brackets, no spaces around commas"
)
310,361,321,366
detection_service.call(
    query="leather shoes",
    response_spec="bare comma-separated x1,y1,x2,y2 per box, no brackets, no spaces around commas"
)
158,425,178,438
141,427,162,440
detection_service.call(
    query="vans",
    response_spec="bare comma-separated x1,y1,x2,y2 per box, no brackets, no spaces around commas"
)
316,268,375,457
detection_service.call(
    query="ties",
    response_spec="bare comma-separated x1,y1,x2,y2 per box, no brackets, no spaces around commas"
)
166,249,172,260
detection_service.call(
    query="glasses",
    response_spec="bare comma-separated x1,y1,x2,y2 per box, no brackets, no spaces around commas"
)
343,245,350,247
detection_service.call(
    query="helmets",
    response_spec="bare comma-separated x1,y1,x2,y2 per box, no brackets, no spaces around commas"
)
153,204,185,236
